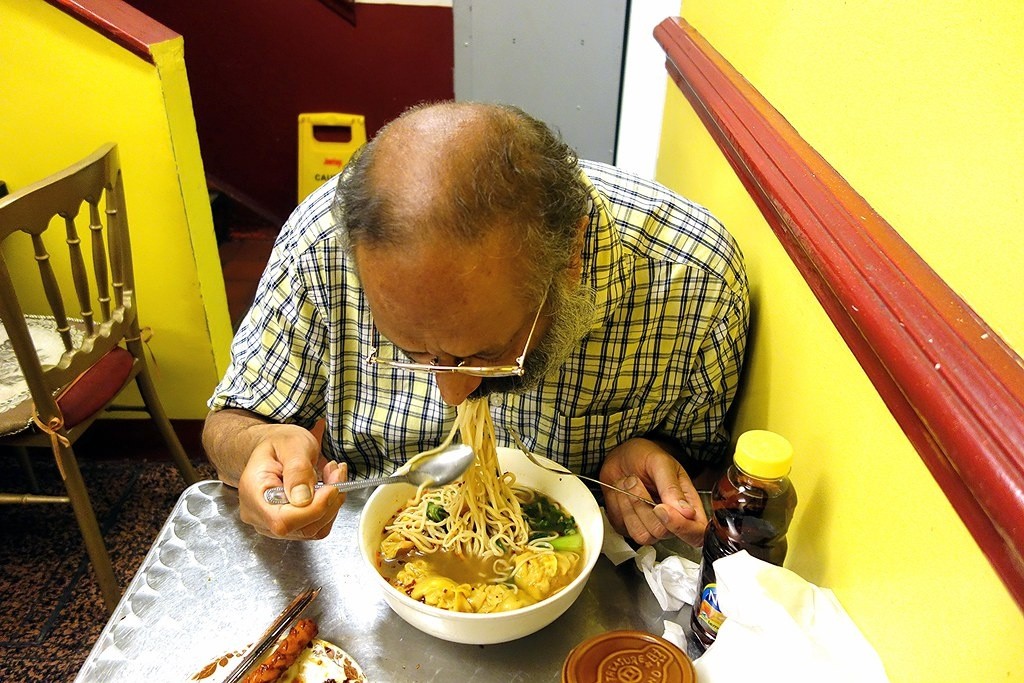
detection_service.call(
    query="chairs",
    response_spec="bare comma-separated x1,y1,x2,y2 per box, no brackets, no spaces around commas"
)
0,141,199,620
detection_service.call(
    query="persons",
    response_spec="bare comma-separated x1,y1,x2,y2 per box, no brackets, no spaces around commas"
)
201,99,749,555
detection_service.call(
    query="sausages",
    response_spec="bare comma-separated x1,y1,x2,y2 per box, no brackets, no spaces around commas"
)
240,616,318,683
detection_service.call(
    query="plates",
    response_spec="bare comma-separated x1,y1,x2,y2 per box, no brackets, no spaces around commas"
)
188,637,368,683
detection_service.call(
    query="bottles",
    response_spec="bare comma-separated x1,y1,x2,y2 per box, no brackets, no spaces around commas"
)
689,430,795,646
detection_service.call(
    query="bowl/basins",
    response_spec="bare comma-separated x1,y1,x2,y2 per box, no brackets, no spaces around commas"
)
357,445,604,645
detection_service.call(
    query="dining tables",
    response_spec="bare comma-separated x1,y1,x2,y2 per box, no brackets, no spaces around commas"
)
70,480,710,683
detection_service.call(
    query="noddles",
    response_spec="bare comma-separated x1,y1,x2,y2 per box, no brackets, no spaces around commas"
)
358,391,587,617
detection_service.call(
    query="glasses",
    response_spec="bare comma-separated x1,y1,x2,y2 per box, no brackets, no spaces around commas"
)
365,264,554,378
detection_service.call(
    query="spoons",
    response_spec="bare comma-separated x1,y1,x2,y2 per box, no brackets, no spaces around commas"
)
260,444,473,504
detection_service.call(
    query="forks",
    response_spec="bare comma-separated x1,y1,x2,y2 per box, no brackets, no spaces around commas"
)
503,420,666,513
